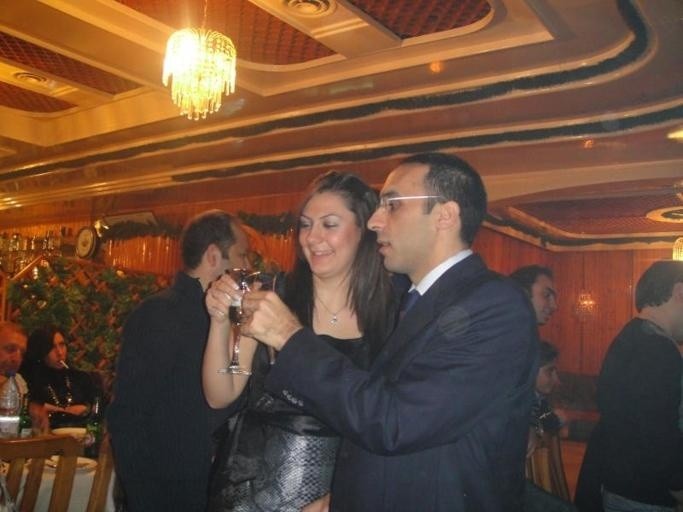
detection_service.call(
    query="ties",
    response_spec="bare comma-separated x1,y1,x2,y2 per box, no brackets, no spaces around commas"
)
398,289,422,324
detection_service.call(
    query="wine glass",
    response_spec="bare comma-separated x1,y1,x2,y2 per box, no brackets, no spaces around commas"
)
239,272,284,367
216,266,254,378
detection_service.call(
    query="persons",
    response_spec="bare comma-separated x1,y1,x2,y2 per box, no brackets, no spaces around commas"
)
507,263,559,326
237,151,539,512
199,168,398,511
107,209,254,512
0,320,50,435
523,340,560,459
596,259,683,512
19,324,95,429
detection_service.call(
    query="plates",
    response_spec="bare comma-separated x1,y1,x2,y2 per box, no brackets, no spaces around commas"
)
0,426,98,471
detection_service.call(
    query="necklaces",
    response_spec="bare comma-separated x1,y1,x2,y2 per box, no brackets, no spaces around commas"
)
316,294,347,325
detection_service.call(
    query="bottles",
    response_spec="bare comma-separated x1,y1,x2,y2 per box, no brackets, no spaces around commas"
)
0,369,22,437
16,396,32,438
84,397,102,457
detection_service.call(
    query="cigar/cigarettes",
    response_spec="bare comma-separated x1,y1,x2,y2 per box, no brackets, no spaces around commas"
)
60,359,69,369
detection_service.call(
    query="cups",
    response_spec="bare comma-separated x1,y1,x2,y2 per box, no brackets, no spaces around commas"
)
0,416,20,436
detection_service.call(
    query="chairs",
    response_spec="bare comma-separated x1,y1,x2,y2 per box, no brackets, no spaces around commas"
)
526,424,571,501
0,433,79,512
87,432,113,511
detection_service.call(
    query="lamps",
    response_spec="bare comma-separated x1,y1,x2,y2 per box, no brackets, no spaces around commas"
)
161,0,236,122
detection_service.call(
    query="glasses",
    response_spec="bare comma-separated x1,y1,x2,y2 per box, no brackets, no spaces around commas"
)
376,195,449,213
